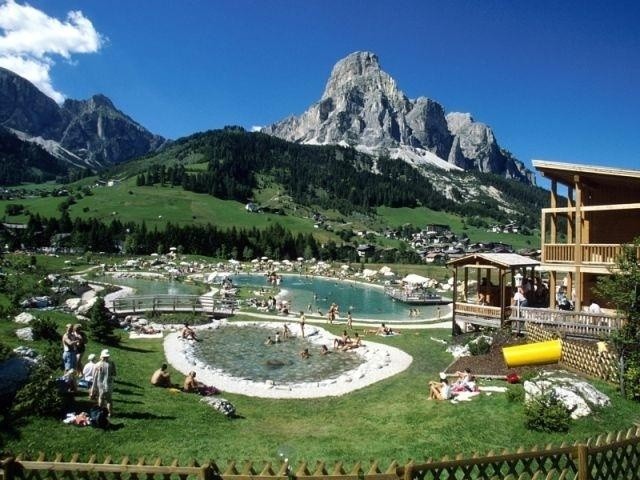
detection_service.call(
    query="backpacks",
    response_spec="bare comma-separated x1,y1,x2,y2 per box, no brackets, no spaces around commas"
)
88,406,109,428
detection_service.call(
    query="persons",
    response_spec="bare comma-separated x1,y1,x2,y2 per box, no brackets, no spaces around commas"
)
182,371,205,391
140,326,155,334
264,337,273,345
414,308,419,317
92,348,117,418
61,322,80,374
299,311,306,338
298,347,312,360
275,332,282,342
149,363,171,387
70,323,88,371
437,307,441,317
319,344,331,356
427,372,452,402
182,321,197,340
477,270,572,310
588,298,603,314
82,353,97,389
408,309,414,318
220,270,291,315
453,375,478,392
306,291,394,352
283,324,291,341
454,367,472,385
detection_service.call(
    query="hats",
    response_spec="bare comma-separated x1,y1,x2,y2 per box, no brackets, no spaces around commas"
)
88,353,94,360
100,348,110,358
